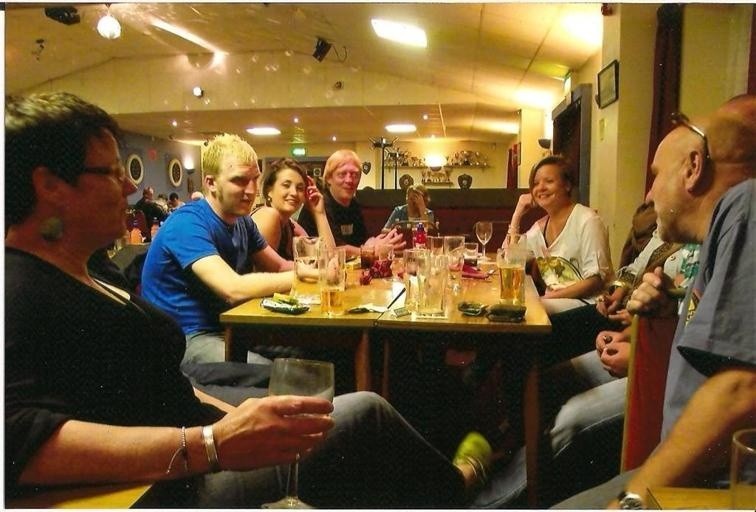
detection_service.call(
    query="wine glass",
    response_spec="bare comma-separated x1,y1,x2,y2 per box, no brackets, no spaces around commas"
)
261,358,334,509
476,222,492,261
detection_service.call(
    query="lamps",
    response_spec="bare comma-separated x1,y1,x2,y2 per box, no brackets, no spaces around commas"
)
311,37,333,62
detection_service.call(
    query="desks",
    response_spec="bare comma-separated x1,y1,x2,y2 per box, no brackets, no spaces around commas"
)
376,253,552,510
218,263,411,391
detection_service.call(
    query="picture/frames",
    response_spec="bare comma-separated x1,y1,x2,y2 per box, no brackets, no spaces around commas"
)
597,59,620,109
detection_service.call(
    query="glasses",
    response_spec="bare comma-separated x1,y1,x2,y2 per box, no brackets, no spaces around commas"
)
664,110,711,164
82,157,125,180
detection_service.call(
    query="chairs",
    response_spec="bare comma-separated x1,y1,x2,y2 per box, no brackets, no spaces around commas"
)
620,287,691,478
132,209,150,237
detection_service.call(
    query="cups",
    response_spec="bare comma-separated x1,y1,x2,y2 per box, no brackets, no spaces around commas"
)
290,237,323,305
444,236,465,288
403,249,430,310
415,255,447,313
317,248,346,316
377,245,394,269
497,248,527,306
431,237,444,255
360,246,375,268
729,428,756,510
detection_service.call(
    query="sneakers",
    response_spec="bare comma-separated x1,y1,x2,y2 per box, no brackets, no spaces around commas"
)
452,431,492,478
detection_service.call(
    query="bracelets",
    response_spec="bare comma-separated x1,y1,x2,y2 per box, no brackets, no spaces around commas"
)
506,225,521,236
607,279,629,297
166,423,191,482
200,424,221,477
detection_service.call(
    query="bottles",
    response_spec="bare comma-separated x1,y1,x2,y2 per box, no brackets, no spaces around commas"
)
415,223,427,249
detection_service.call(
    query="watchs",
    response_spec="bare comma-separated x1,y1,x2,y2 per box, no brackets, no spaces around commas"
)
612,489,648,510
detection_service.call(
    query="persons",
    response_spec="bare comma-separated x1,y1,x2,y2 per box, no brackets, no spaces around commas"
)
247,157,336,278
191,191,203,201
538,223,702,359
382,183,435,236
499,153,616,472
298,149,407,263
158,194,168,203
136,187,154,222
141,130,335,363
169,193,185,209
5,91,496,509
522,90,756,493
178,361,277,406
549,115,756,510
